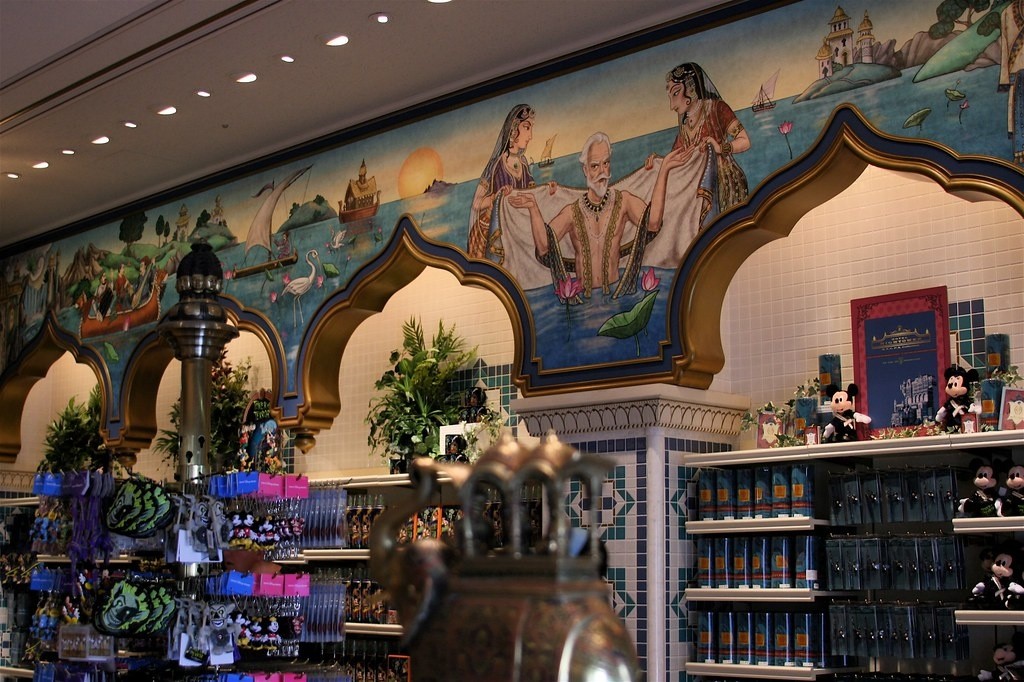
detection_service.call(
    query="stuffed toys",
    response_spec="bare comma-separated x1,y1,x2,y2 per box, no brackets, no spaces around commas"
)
822,383,871,443
225,511,276,552
934,368,979,433
440,436,470,463
958,460,1024,519
977,641,1024,682
227,611,282,650
466,388,492,422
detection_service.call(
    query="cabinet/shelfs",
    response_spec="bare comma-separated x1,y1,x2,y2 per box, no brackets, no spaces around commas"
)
298,470,454,635
685,427,1024,682
0,496,174,682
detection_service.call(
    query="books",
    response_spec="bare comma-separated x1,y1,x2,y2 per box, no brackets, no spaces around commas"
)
967,551,1024,611
